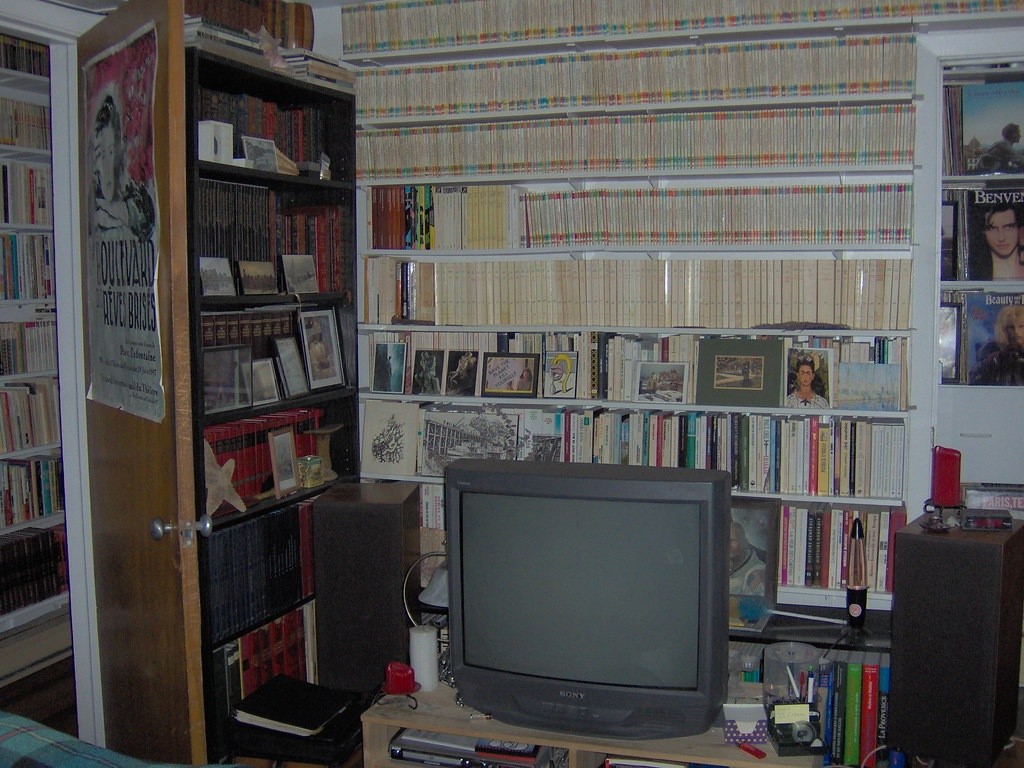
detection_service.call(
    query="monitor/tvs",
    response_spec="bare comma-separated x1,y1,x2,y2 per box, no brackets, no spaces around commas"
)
444,458,732,740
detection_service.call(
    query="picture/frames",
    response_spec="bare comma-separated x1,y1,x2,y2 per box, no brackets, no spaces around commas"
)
251,359,282,407
270,333,312,401
296,308,346,395
481,352,540,398
729,495,781,607
199,343,254,415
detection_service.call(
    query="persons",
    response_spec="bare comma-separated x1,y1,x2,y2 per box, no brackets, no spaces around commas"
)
970,205,1024,279
963,301,1023,387
730,521,766,596
974,123,1024,174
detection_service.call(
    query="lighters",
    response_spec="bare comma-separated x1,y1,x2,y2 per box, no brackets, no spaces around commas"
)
736,740,767,759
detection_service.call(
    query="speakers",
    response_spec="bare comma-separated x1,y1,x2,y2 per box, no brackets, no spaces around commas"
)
886,506,1024,768
312,483,421,708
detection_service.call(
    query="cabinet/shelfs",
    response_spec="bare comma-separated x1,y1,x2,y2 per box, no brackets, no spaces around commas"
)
0,2,1024,768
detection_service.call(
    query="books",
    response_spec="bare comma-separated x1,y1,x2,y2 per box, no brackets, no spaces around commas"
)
185,16,358,87
362,399,905,500
780,504,907,591
203,408,328,519
360,477,445,529
604,754,689,768
941,289,1024,386
363,256,913,332
388,729,546,768
818,658,890,768
201,306,297,358
199,496,363,768
196,87,349,294
0,33,69,616
370,331,909,411
341,0,1024,250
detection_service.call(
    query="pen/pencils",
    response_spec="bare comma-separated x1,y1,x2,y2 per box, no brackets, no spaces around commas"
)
785,660,815,703
